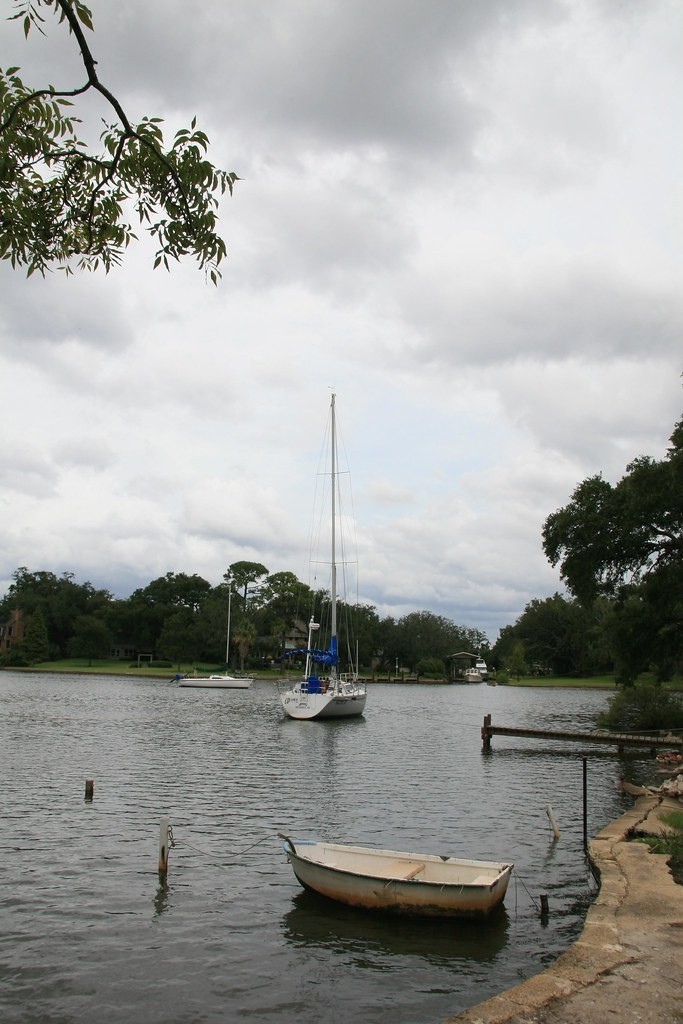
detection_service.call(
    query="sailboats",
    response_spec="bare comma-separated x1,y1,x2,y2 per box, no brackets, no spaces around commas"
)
167,580,257,690
274,385,369,721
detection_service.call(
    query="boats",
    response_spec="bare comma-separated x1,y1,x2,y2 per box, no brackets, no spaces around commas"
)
276,831,514,924
463,667,483,684
